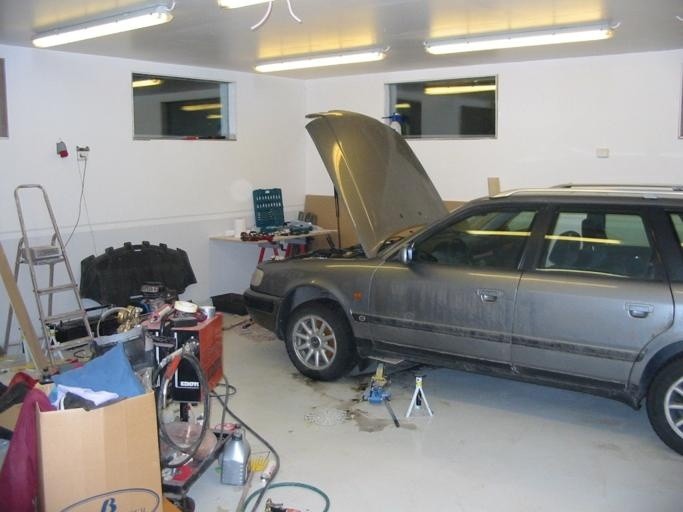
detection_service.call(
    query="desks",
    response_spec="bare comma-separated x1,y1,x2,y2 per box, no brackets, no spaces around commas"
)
210,227,339,270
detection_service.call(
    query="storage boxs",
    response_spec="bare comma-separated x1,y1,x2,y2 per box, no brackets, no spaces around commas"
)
210,292,249,316
0,382,163,512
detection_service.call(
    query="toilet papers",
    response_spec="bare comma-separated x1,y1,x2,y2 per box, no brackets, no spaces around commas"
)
234,218,245,238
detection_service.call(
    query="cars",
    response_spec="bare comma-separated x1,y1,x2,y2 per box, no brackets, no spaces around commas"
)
244,110,683,455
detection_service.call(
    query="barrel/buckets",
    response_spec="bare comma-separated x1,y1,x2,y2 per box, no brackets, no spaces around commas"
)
221,430,251,485
92,307,145,344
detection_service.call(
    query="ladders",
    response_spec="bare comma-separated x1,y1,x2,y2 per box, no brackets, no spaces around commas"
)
4,184,94,367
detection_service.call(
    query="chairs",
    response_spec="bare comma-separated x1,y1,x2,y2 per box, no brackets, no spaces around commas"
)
548,217,611,269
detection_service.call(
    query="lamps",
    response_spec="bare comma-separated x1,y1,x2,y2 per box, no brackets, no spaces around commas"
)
253,42,391,74
423,22,612,57
29,6,173,48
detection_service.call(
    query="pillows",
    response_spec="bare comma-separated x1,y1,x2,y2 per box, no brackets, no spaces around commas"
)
51,340,147,404
